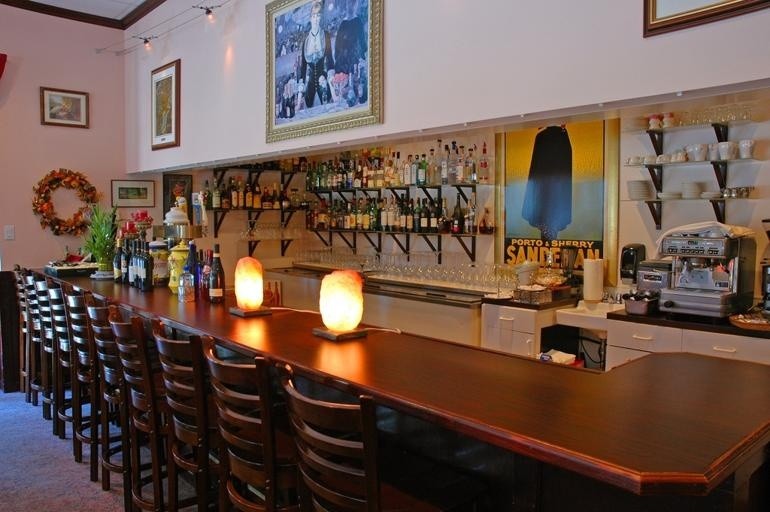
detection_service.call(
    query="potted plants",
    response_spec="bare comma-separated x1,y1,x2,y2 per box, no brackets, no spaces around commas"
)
83,204,121,280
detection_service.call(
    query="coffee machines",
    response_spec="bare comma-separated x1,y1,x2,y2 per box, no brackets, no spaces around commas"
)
658,221,758,318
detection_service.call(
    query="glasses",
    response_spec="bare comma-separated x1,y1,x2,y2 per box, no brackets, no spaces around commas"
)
311,13,321,17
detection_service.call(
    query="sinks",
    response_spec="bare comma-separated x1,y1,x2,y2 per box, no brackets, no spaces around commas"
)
556,301,625,331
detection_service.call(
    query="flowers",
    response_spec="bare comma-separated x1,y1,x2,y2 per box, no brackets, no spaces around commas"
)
32,168,101,237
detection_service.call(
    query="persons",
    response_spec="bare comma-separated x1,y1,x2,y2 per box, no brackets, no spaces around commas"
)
299,0,334,108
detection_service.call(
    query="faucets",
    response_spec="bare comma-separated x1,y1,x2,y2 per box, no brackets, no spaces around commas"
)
610,293,622,304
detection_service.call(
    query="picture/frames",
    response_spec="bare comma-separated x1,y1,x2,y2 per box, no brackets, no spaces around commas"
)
491,117,623,291
149,58,182,151
163,174,194,226
38,85,91,130
642,0,769,39
110,179,156,209
264,1,385,143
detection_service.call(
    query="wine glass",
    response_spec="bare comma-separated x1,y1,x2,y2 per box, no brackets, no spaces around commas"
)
366,245,516,290
240,219,303,242
296,240,374,273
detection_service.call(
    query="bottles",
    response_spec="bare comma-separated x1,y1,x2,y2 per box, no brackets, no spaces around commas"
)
203,177,307,209
113,236,156,293
176,240,225,306
308,137,492,190
63,245,69,257
303,193,493,235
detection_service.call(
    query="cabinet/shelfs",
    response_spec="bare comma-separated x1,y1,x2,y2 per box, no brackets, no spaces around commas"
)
481,302,566,358
620,119,764,228
683,328,770,364
207,164,305,256
605,319,684,374
306,182,493,267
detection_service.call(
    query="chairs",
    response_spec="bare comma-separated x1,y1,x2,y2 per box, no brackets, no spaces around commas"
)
276,362,441,511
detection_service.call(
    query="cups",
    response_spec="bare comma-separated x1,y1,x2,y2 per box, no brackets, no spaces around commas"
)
685,138,755,161
622,149,689,166
678,105,754,127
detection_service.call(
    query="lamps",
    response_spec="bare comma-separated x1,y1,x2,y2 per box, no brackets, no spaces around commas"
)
228,255,274,318
131,1,222,51
311,269,369,343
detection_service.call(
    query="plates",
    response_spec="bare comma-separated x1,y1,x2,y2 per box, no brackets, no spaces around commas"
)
728,310,770,332
701,191,723,199
626,178,651,201
681,180,702,199
655,190,681,200
